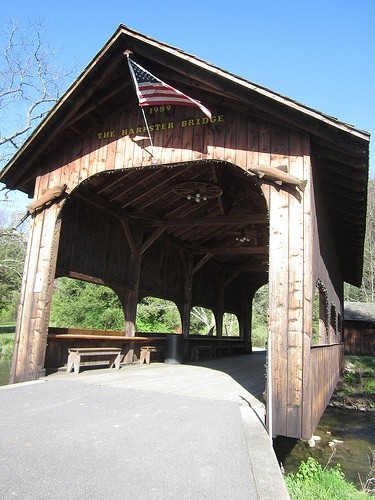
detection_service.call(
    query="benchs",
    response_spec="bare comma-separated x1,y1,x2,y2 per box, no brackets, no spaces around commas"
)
67,347,122,374
140,346,168,365
190,344,244,362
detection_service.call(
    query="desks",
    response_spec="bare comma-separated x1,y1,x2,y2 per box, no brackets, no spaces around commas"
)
47,333,244,343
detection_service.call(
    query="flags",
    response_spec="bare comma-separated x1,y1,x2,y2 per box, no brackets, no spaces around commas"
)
126,58,212,120
